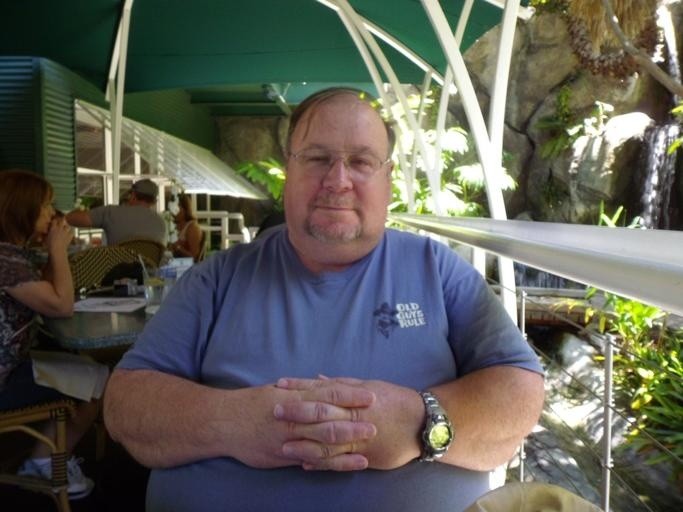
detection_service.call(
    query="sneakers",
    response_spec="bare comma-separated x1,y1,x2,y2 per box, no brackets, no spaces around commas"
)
16,456,94,500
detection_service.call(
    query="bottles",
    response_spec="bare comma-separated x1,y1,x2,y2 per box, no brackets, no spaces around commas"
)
158,251,177,301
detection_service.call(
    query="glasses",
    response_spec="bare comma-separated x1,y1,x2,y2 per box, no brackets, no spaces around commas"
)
288,145,390,182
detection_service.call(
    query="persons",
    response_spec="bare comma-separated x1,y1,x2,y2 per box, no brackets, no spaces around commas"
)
64,177,167,256
99,85,546,511
0,170,97,501
167,188,205,259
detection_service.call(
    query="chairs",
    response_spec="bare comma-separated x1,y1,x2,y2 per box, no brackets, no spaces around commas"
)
0,231,215,512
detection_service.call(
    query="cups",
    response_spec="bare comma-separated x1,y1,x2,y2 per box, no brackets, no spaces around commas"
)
143,269,165,314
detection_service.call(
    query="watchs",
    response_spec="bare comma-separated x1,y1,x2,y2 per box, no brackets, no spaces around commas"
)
415,387,456,462
